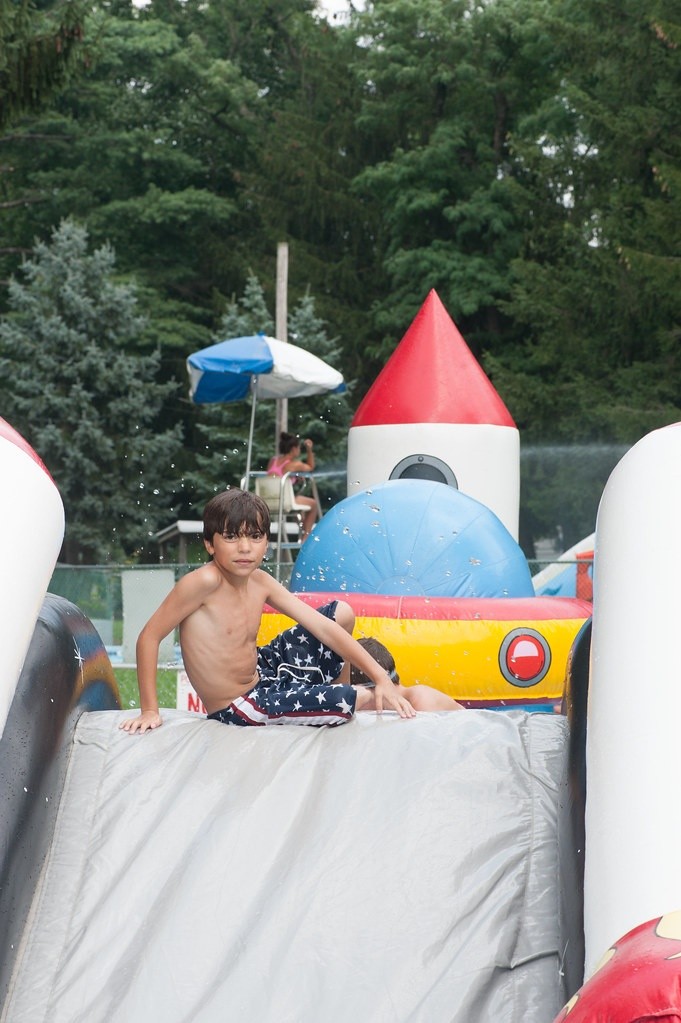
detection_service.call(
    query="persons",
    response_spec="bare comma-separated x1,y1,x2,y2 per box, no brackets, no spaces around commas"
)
267,431,319,543
117,491,417,735
351,637,466,711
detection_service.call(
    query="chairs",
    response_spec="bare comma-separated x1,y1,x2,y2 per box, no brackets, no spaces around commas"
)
245,471,323,584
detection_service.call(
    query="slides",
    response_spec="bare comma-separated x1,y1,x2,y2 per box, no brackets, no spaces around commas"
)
0,706,572,1023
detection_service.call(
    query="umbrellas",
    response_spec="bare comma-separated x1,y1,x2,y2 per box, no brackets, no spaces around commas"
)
186,334,346,490
